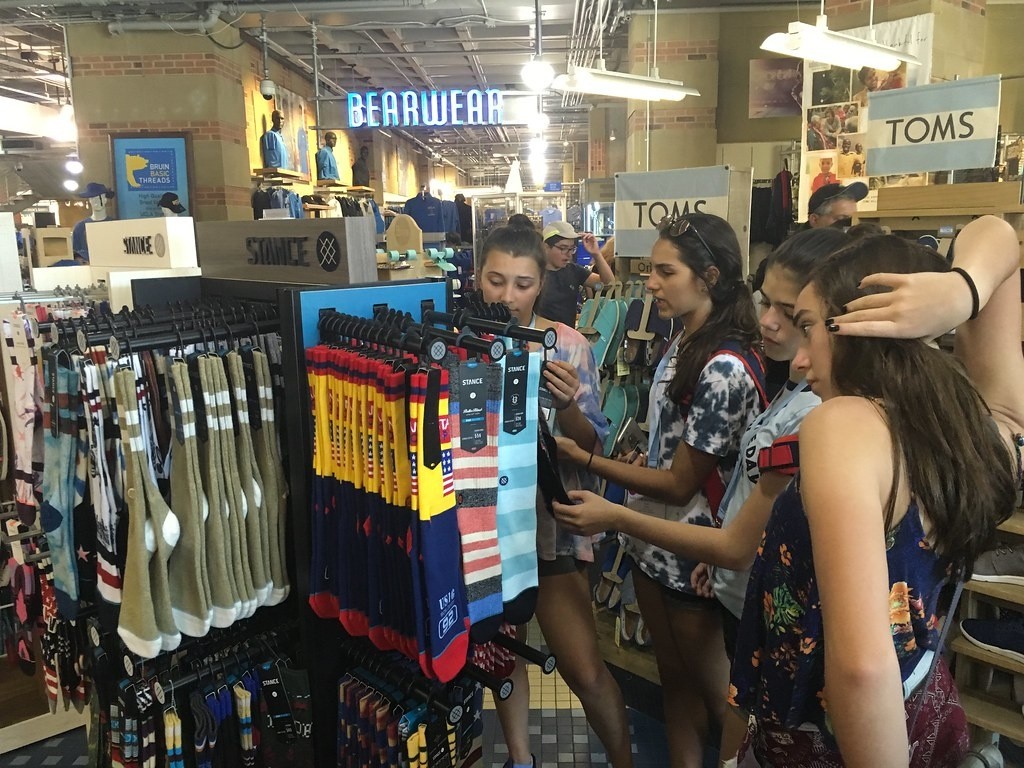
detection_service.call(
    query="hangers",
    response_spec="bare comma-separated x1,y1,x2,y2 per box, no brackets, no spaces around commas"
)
352,192,374,201
19,296,519,372
752,177,773,190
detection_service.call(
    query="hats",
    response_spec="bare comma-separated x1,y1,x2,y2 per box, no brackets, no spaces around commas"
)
808,182,868,214
79,183,108,198
542,221,581,242
158,192,186,214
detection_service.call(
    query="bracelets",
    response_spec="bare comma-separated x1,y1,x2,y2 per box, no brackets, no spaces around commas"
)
586,453,593,470
950,267,980,321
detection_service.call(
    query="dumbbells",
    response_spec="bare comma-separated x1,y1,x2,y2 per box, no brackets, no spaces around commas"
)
388,250,417,261
426,248,454,260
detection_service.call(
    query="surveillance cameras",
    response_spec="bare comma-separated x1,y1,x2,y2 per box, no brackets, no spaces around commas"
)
260,81,276,100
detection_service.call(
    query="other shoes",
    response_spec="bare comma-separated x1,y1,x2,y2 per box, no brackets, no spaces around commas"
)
575,294,696,649
939,504,1023,768
503,752,536,768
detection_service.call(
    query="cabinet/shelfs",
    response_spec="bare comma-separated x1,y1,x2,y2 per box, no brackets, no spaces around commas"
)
947,514,1024,740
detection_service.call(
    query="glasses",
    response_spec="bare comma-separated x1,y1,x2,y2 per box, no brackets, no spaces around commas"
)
655,215,719,268
551,243,578,256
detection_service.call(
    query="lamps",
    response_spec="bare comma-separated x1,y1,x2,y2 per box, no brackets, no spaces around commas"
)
759,0,922,72
551,0,701,102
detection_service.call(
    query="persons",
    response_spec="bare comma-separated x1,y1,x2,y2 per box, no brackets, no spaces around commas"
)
443,216,615,330
261,110,289,170
157,193,187,217
553,212,768,768
351,146,370,187
806,65,903,194
316,131,341,180
71,182,116,266
476,214,634,768
553,220,883,768
728,216,1023,768
